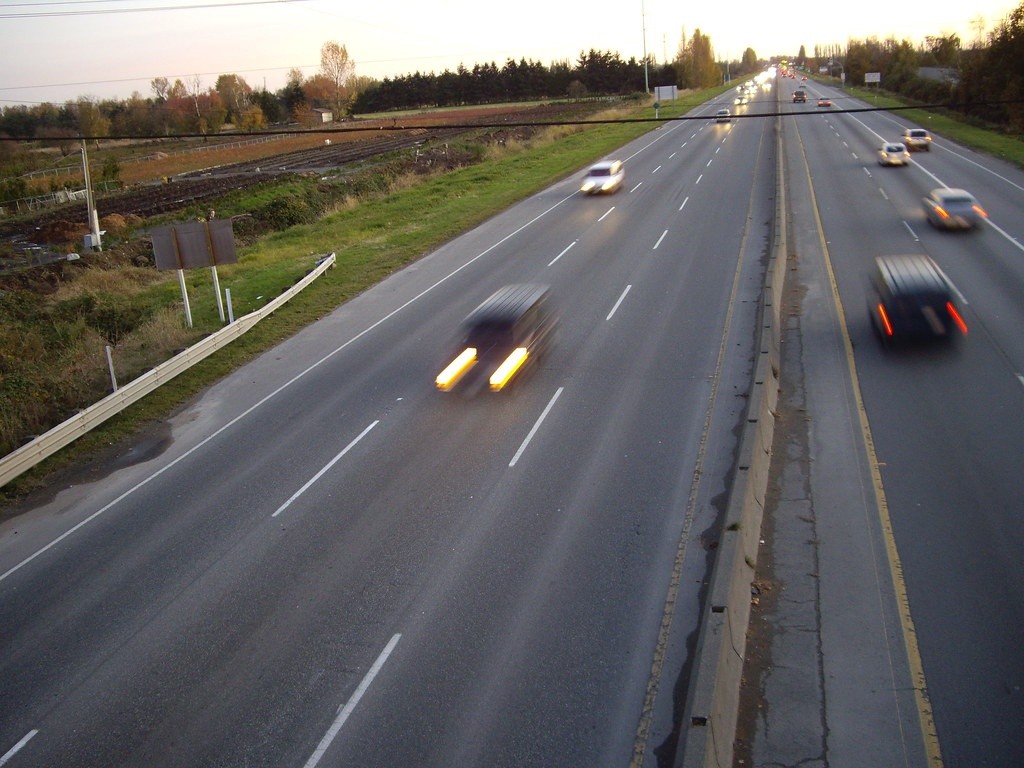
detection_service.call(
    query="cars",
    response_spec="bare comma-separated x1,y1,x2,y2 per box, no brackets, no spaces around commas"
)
817,96,832,107
801,75,808,81
733,61,798,106
716,109,732,123
798,82,807,89
878,142,911,168
922,186,983,235
792,90,807,103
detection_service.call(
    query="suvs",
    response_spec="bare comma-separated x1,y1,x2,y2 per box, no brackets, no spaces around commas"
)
440,281,554,390
901,128,932,152
581,159,625,196
865,253,961,348
